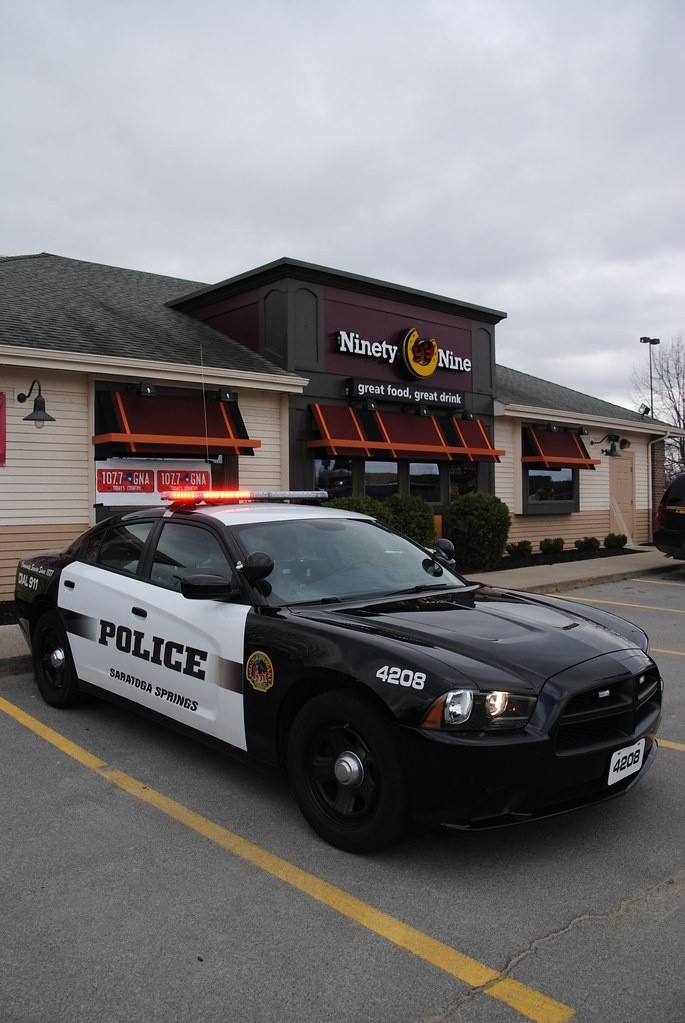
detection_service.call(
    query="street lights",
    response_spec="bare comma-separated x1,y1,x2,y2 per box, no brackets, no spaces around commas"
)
640,337,661,419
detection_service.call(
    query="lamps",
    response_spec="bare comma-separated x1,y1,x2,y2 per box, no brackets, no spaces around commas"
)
607,434,620,442
360,399,379,411
590,433,624,457
528,420,558,432
414,404,433,417
574,427,589,435
620,438,631,449
638,403,650,419
17,379,56,429
215,388,235,401
457,408,477,421
139,381,156,397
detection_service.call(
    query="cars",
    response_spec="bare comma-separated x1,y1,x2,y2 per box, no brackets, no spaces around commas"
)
15,491,666,858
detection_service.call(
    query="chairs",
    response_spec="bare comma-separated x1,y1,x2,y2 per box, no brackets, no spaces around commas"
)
270,535,303,583
174,530,213,575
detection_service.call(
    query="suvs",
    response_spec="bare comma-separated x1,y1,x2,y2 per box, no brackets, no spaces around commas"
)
652,474,685,561
